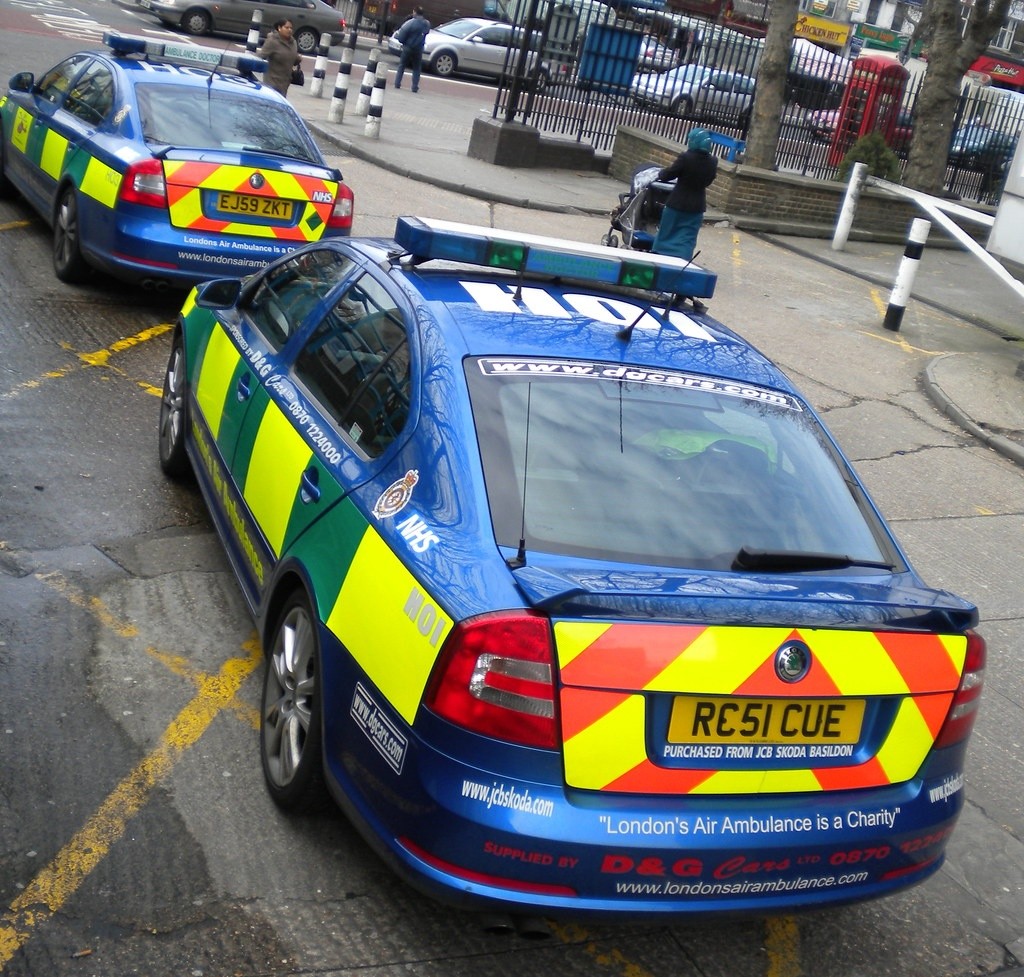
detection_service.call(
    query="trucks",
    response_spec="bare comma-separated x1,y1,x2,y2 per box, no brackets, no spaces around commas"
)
361,0,513,37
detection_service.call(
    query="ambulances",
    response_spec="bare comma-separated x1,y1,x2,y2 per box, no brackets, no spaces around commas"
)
0,31,354,281
158,215,988,913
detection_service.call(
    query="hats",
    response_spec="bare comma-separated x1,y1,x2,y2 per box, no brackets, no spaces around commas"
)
689,128,711,151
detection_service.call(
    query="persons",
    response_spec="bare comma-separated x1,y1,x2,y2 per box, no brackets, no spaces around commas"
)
650,128,718,296
259,18,303,101
395,7,430,92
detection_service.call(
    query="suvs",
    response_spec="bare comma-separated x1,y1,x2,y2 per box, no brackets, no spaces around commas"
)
389,17,553,93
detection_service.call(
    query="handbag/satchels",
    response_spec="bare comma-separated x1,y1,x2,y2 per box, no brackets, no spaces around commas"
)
290,64,304,86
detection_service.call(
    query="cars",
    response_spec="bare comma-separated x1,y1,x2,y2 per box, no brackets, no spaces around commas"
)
630,63,756,125
950,126,1018,175
807,100,911,172
135,0,346,53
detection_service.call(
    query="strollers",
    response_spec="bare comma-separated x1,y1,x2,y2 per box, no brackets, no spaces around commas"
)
602,163,678,252
